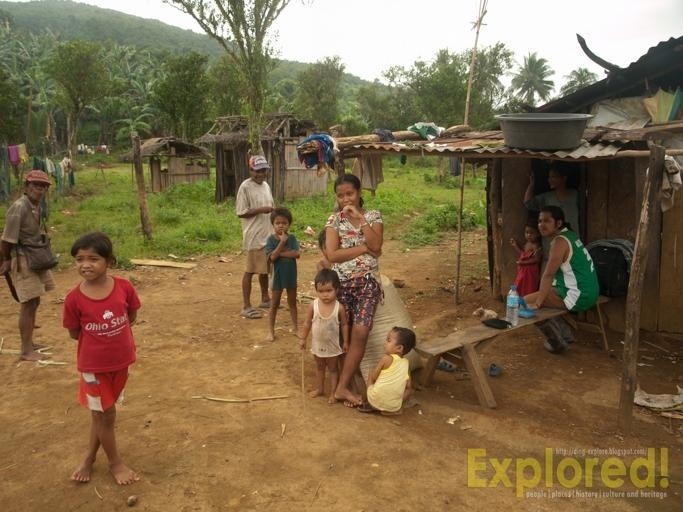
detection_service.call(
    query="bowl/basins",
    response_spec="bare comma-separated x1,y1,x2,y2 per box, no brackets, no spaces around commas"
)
490,110,598,152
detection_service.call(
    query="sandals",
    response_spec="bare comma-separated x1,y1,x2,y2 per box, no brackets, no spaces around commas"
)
488,363,497,376
439,361,454,371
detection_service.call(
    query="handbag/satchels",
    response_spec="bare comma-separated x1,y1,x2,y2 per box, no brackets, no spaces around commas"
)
21,232,59,270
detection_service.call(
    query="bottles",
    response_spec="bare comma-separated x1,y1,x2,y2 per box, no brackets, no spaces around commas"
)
505,285,520,327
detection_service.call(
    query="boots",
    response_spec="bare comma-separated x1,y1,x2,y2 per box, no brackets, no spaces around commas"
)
536,317,575,353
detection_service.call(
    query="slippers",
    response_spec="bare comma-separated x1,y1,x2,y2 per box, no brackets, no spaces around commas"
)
260,301,283,309
243,308,262,318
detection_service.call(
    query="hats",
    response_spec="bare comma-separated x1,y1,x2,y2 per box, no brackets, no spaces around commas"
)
249,155,270,170
26,170,52,185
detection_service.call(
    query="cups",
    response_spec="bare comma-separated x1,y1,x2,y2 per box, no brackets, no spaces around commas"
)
304,225,315,236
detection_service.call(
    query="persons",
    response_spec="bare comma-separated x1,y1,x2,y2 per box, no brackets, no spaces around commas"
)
234,153,416,417
0,169,60,361
62,231,142,486
508,163,601,354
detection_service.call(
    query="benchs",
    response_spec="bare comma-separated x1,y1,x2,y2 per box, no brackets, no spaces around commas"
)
413,295,611,409
